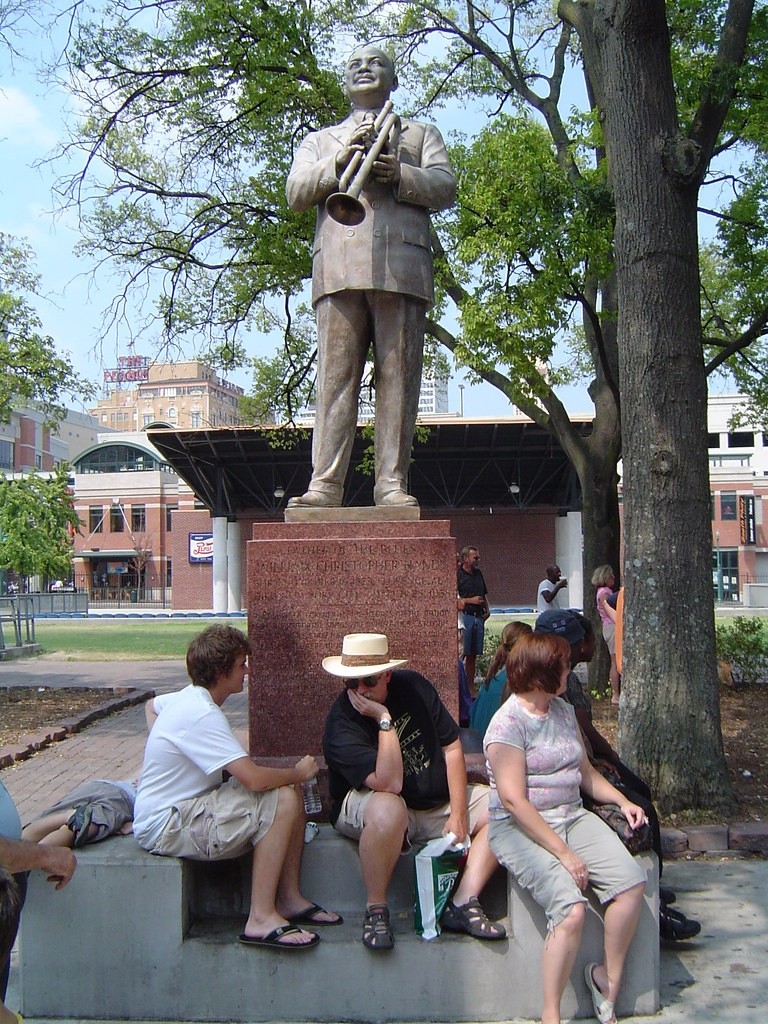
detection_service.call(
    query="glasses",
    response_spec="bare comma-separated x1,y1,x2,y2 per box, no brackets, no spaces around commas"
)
343,675,381,690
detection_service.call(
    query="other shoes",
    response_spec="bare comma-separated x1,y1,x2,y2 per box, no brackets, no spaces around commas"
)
658,903,701,940
658,883,678,905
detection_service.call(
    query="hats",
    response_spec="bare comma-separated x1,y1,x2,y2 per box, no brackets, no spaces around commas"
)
321,633,409,679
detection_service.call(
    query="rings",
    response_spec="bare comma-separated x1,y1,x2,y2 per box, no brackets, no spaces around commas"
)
579,874,583,878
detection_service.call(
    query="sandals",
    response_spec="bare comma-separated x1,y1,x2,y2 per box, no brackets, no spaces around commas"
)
438,895,508,939
361,903,395,950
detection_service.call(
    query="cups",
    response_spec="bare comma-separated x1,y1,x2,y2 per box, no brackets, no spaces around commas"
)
559,577,566,585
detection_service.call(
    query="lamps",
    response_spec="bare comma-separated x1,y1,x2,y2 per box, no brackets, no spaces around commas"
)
508,482,520,492
274,486,285,497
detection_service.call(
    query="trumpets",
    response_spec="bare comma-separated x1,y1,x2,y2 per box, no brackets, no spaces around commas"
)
325,100,402,226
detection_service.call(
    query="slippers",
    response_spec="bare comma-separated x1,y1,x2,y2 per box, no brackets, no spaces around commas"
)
287,901,344,925
239,921,322,949
584,962,618,1024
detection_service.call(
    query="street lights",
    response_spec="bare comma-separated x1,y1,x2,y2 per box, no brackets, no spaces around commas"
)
716,528,723,602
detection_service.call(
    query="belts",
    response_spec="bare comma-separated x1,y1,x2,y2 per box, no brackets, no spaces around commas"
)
462,610,485,618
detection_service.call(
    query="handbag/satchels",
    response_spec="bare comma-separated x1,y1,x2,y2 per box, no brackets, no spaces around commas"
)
412,833,471,941
583,800,653,856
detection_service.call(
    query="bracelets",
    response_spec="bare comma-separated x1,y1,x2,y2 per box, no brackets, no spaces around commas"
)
486,610,490,613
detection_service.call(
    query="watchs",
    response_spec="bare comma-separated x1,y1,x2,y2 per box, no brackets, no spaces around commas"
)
378,720,394,730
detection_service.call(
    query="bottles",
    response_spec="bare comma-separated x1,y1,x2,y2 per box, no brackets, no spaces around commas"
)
301,776,322,814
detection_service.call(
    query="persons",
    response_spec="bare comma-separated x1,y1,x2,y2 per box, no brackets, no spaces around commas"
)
459,632,701,1024
288,47,456,507
465,618,533,754
533,564,700,941
0,998,24,1024
21,776,140,847
323,634,508,951
0,780,77,1004
133,622,344,949
455,545,493,728
